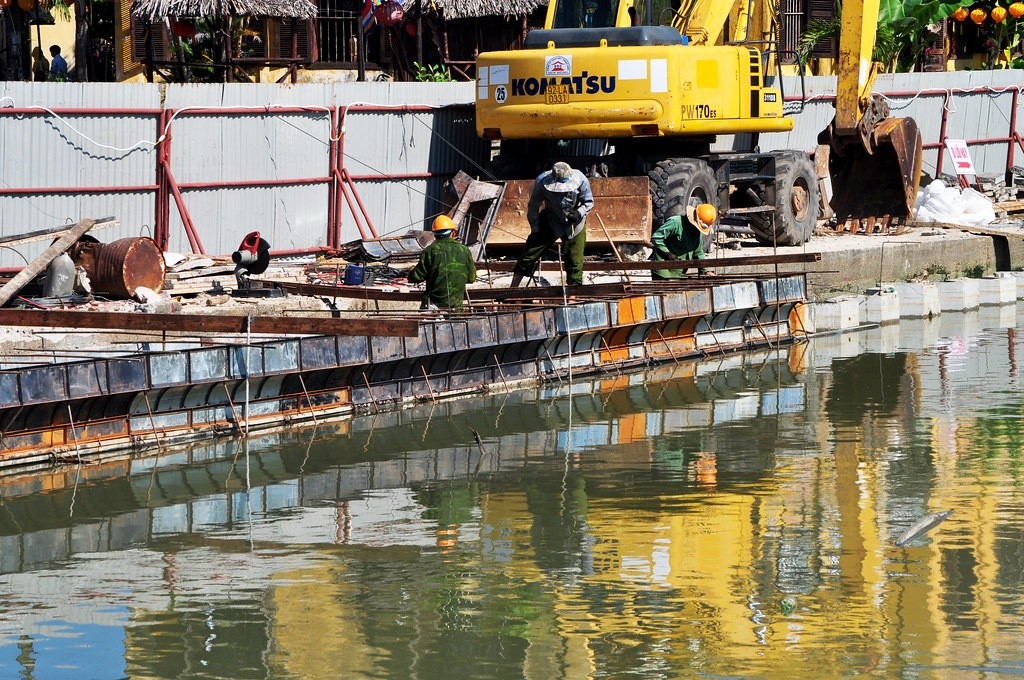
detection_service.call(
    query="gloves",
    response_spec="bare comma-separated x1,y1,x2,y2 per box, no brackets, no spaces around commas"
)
566,210,583,223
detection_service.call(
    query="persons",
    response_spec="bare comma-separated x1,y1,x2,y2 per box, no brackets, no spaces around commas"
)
408,215,476,309
651,204,716,279
49,45,67,77
510,162,594,286
30,46,49,81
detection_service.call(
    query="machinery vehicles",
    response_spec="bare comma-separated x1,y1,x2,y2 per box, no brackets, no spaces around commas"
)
441,0,922,258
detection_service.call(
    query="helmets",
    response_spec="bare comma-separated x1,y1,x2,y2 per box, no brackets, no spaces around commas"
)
432,215,455,231
696,204,716,233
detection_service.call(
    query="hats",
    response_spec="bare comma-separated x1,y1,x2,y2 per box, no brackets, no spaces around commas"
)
686,205,714,235
543,162,583,192
